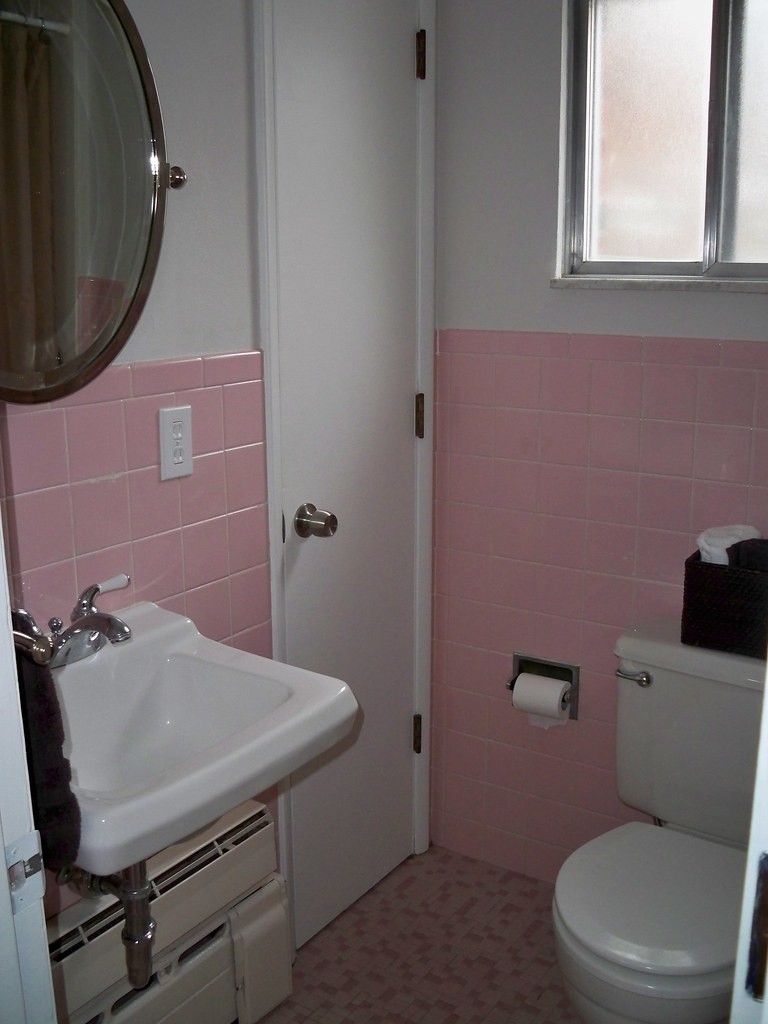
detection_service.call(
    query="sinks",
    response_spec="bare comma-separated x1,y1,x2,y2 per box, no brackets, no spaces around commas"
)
36,601,360,880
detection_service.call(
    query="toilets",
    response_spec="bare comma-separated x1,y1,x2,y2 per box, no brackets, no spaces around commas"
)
548,606,768,1023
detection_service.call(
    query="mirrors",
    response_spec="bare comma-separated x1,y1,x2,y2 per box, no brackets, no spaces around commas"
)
0,0,189,405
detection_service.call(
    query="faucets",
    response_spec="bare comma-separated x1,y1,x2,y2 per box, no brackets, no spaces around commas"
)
54,609,133,662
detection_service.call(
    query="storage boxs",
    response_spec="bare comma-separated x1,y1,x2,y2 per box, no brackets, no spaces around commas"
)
679,536,768,661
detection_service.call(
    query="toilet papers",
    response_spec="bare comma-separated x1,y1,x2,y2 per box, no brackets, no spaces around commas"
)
512,670,574,729
